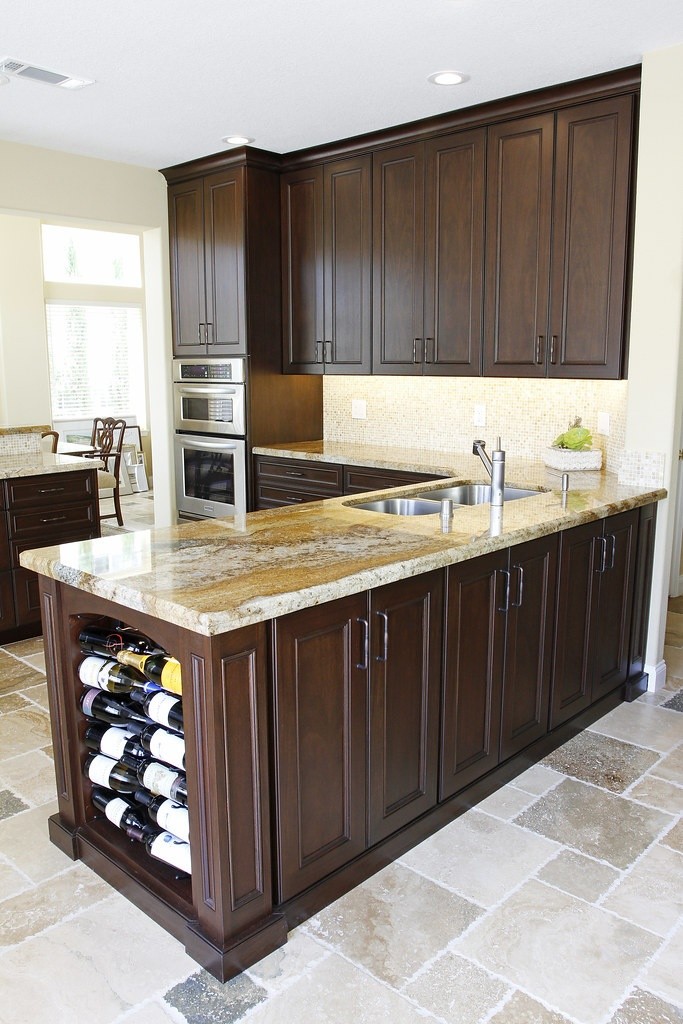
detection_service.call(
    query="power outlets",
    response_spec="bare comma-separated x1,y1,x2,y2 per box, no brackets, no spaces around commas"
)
473,403,488,426
598,411,610,439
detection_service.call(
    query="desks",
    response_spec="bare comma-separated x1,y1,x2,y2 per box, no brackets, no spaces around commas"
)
57,439,103,458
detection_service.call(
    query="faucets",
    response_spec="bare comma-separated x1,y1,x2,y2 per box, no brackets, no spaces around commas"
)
472,436,505,507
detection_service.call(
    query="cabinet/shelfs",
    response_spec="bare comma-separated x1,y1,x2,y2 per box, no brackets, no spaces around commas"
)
0,469,102,645
272,566,444,906
373,123,488,376
343,464,450,496
440,529,558,804
254,454,343,507
627,500,659,680
158,144,249,356
485,91,638,378
38,573,272,930
279,153,373,376
548,505,639,731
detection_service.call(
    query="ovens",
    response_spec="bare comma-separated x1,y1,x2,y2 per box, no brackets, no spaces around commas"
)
171,353,253,522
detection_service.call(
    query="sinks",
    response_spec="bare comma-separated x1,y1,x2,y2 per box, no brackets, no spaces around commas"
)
415,480,552,505
342,496,464,516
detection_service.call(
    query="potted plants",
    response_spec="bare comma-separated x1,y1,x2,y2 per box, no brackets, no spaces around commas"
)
544,417,602,472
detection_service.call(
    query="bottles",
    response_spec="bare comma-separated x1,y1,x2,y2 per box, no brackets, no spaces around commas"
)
76,613,188,878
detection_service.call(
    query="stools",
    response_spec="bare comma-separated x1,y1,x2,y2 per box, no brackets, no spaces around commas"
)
86,417,127,527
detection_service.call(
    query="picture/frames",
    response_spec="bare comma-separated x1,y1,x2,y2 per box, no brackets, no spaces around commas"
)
82,426,144,499
